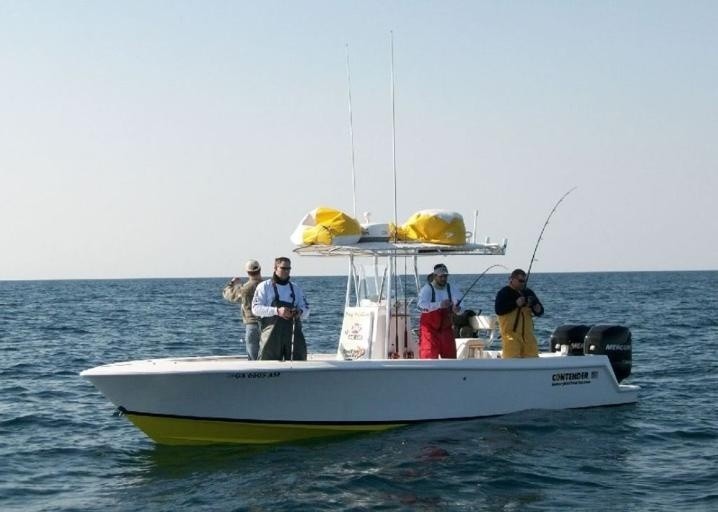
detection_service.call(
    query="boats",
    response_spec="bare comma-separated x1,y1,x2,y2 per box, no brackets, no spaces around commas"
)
82,18,642,448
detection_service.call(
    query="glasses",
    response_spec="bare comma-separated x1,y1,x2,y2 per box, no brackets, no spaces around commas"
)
515,278,525,283
280,266,291,270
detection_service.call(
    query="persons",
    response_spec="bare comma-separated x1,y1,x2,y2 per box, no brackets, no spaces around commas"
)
251,258,310,360
494,269,544,358
223,260,266,360
418,264,464,358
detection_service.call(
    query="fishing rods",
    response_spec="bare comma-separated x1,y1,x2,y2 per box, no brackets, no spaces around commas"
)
453,263,511,307
290,314,297,358
512,185,577,334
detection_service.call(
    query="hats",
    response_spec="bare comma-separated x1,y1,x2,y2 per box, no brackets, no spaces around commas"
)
434,267,448,277
246,260,260,272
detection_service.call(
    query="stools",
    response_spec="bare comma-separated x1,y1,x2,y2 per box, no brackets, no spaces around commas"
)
469,345,485,359
